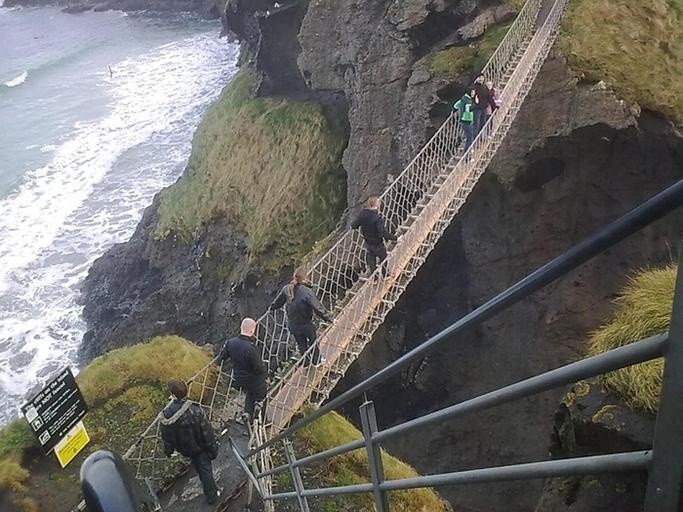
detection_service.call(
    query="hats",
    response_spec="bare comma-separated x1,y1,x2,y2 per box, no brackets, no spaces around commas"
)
168,377,190,399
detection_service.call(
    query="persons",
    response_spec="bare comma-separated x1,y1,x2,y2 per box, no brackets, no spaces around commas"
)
221,317,274,428
350,196,404,285
452,73,502,163
159,378,222,505
271,266,335,368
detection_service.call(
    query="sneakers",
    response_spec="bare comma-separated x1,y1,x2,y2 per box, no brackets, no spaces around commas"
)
301,352,326,370
241,409,273,430
371,271,392,286
